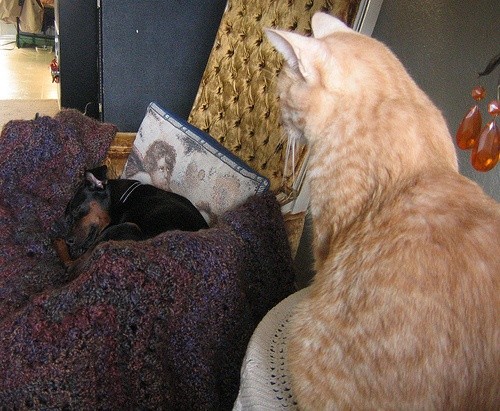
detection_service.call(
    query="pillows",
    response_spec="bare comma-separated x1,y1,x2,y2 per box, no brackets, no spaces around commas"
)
120,101,270,231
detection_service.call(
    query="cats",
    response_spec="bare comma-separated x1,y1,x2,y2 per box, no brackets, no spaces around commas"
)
263,11,499,410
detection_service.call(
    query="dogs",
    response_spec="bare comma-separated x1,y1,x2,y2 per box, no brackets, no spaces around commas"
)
53,164,211,283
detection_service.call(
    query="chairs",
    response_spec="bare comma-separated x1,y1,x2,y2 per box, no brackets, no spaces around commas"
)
0,0,384,410
